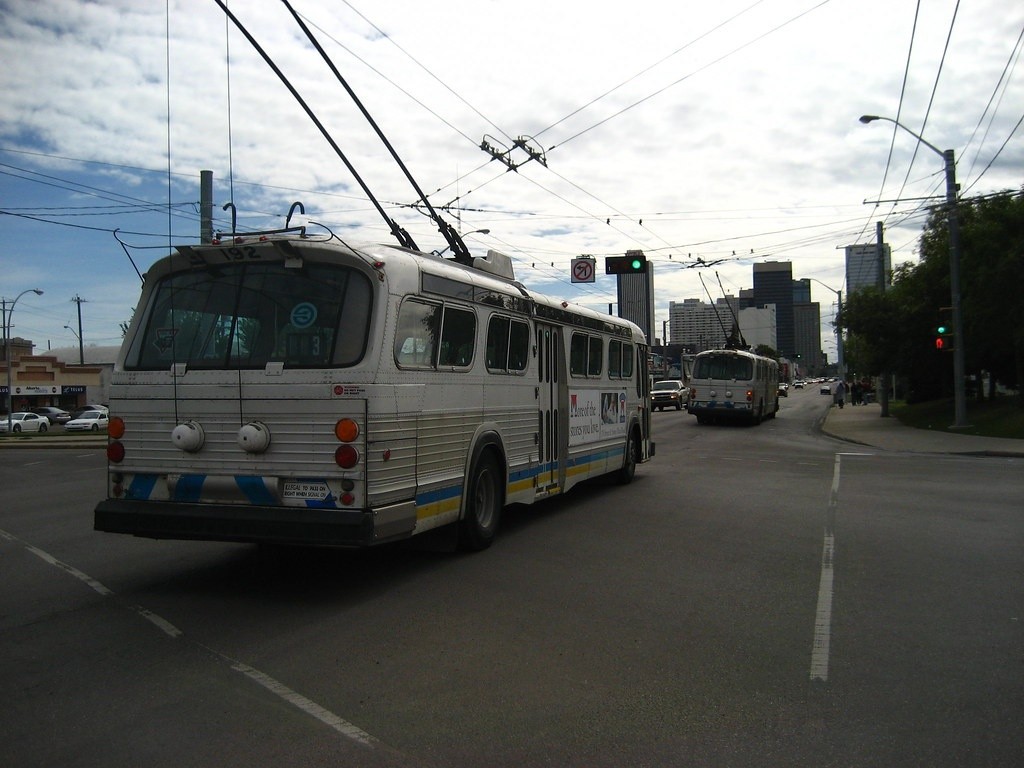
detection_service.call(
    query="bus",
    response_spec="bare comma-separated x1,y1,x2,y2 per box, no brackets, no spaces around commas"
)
688,348,780,425
93,228,656,551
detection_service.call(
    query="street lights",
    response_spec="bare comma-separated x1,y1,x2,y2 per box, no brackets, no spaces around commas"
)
860,116,965,425
6,289,44,432
64,326,84,365
801,278,844,382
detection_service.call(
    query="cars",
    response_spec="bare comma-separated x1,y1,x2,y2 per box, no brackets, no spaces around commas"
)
65,410,109,432
795,381,803,388
801,378,838,385
778,382,788,397
0,412,50,433
69,404,108,419
821,386,831,394
31,407,71,425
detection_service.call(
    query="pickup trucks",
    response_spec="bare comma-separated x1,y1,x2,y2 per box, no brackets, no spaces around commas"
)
650,380,689,412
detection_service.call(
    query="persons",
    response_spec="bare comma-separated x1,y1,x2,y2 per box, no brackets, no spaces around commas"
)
832,380,868,409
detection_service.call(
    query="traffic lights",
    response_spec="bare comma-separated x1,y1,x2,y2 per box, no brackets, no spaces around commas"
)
605,256,646,274
935,307,953,351
